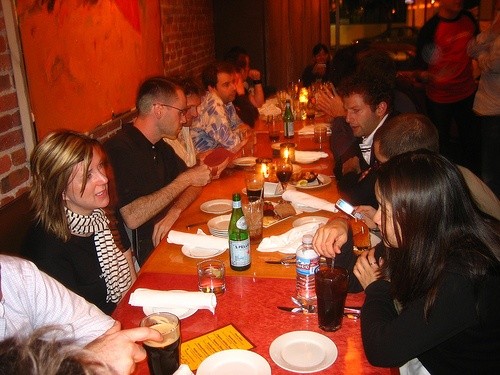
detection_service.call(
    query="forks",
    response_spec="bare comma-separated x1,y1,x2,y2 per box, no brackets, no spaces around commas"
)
290,296,359,319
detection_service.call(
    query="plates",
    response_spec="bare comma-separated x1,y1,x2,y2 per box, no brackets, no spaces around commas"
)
271,164,303,174
231,157,257,166
200,199,232,214
143,290,199,320
196,349,271,375
269,330,338,373
295,156,321,164
288,173,332,188
207,215,231,239
181,245,227,259
263,201,329,228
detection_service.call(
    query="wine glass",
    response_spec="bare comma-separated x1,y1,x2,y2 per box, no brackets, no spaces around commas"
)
275,157,293,204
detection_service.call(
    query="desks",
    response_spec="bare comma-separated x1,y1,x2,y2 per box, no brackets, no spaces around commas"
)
109,93,400,375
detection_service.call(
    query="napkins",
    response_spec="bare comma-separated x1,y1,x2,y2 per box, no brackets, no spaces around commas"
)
167,230,232,250
282,189,340,214
254,221,325,253
293,150,329,160
257,104,282,114
128,288,217,315
298,123,334,134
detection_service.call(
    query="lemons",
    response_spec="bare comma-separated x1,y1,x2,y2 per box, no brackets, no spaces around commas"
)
296,179,308,186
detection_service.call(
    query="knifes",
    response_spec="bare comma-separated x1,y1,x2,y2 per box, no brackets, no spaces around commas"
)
276,306,362,312
265,260,296,265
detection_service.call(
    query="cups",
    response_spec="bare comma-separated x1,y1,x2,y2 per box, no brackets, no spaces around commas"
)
314,263,351,332
139,311,181,375
241,196,263,245
196,259,226,298
240,79,329,200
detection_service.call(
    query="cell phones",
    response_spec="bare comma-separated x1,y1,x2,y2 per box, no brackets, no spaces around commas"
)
335,198,365,222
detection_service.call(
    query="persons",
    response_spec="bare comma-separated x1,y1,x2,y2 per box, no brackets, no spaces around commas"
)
312,148,500,375
184,62,253,153
0,321,114,375
221,47,264,129
317,47,420,183
102,77,213,269
167,74,231,180
333,71,461,210
353,113,500,229
303,44,344,88
416,0,500,175
15,129,138,316
0,254,164,375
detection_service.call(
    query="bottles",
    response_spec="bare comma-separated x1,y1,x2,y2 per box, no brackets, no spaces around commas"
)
228,192,251,271
283,99,295,139
295,234,320,301
271,142,298,149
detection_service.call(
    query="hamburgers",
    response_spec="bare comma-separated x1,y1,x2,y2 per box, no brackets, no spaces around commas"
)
349,220,371,256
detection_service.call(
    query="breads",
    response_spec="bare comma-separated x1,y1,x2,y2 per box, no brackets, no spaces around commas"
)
273,203,296,218
263,216,278,227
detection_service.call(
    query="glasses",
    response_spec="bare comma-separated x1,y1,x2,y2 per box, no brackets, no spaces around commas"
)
153,103,188,117
